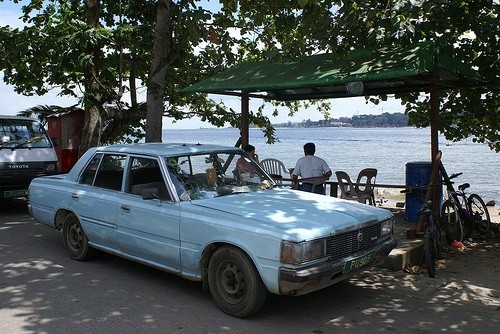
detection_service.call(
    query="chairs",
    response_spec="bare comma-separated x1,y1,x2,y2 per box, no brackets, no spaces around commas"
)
260,158,292,187
335,171,371,205
356,168,377,206
132,182,168,198
294,175,330,195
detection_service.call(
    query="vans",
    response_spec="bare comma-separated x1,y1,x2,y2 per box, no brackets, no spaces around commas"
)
0,115,60,200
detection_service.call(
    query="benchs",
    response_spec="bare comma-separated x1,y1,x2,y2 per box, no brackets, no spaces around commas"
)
86,166,161,191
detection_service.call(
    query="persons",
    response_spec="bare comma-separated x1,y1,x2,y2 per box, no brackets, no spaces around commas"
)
291,143,332,194
236,144,261,179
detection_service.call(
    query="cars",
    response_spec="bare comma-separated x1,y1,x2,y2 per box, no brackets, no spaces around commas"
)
25,142,398,318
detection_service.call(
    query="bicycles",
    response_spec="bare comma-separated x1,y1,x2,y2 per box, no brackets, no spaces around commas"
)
439,172,490,246
400,184,442,278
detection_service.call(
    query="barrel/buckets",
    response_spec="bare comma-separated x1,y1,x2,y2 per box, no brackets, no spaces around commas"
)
405,160,442,222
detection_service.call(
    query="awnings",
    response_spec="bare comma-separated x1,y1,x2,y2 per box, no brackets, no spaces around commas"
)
176,38,489,102
